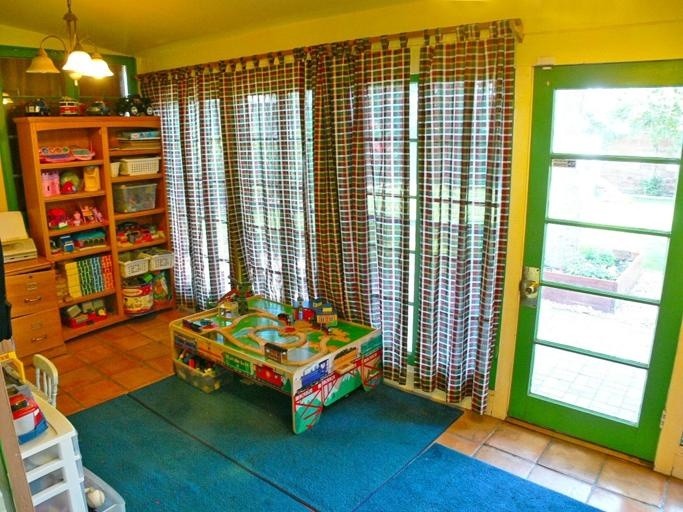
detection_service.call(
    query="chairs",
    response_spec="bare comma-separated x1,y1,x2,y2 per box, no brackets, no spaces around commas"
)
0,351,58,409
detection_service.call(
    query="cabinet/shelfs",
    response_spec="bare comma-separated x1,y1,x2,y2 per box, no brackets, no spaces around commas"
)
1,363,126,512
4,257,69,359
14,117,175,342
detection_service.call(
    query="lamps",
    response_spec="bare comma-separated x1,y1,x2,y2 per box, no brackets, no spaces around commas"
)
25,0,114,86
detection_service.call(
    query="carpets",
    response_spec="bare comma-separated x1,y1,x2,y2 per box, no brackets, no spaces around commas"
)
66,374,606,512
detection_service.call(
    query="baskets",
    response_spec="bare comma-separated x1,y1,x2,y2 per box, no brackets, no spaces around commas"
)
118,252,151,279
110,161,121,179
139,248,175,272
113,157,162,176
